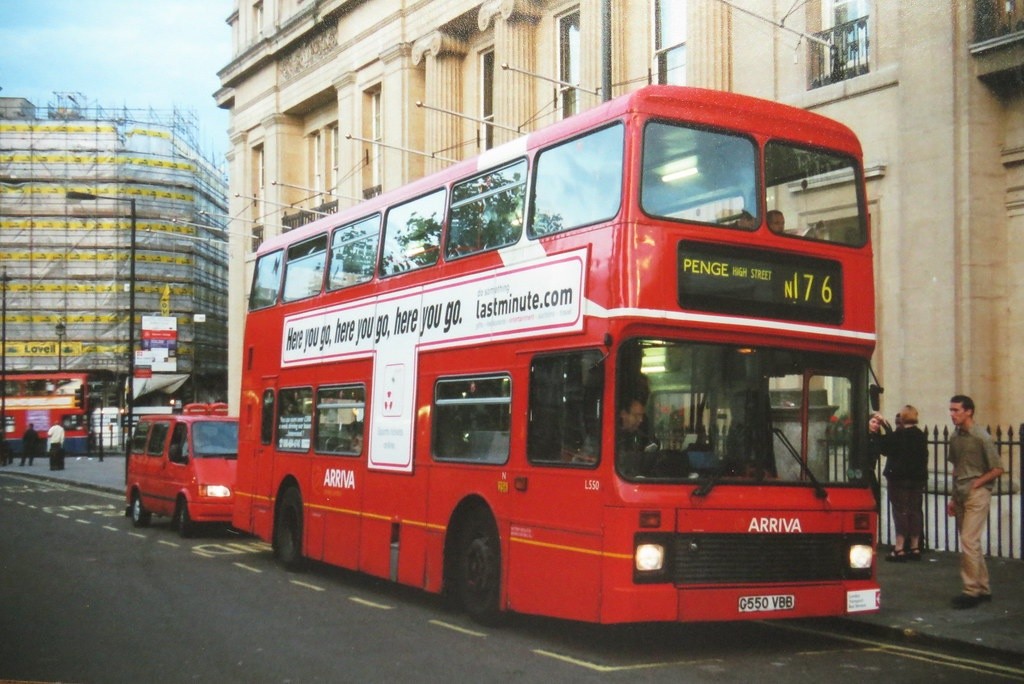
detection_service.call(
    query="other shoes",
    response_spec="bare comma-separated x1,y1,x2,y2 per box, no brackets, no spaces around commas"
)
951,593,993,607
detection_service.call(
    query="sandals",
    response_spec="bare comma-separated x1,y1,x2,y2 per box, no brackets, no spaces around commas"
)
908,548,922,560
885,548,907,562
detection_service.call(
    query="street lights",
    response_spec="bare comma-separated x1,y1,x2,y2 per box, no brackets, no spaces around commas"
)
55,322,66,371
66,190,135,439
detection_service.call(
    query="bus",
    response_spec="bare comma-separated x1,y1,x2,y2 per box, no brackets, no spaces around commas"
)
232,83,897,627
0,373,90,457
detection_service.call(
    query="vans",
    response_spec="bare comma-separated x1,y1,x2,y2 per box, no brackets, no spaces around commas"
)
126,414,238,537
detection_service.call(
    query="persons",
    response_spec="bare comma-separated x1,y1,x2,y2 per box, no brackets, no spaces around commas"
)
947,395,1005,609
618,397,647,449
47,420,65,467
869,404,929,562
19,423,40,466
768,210,786,233
